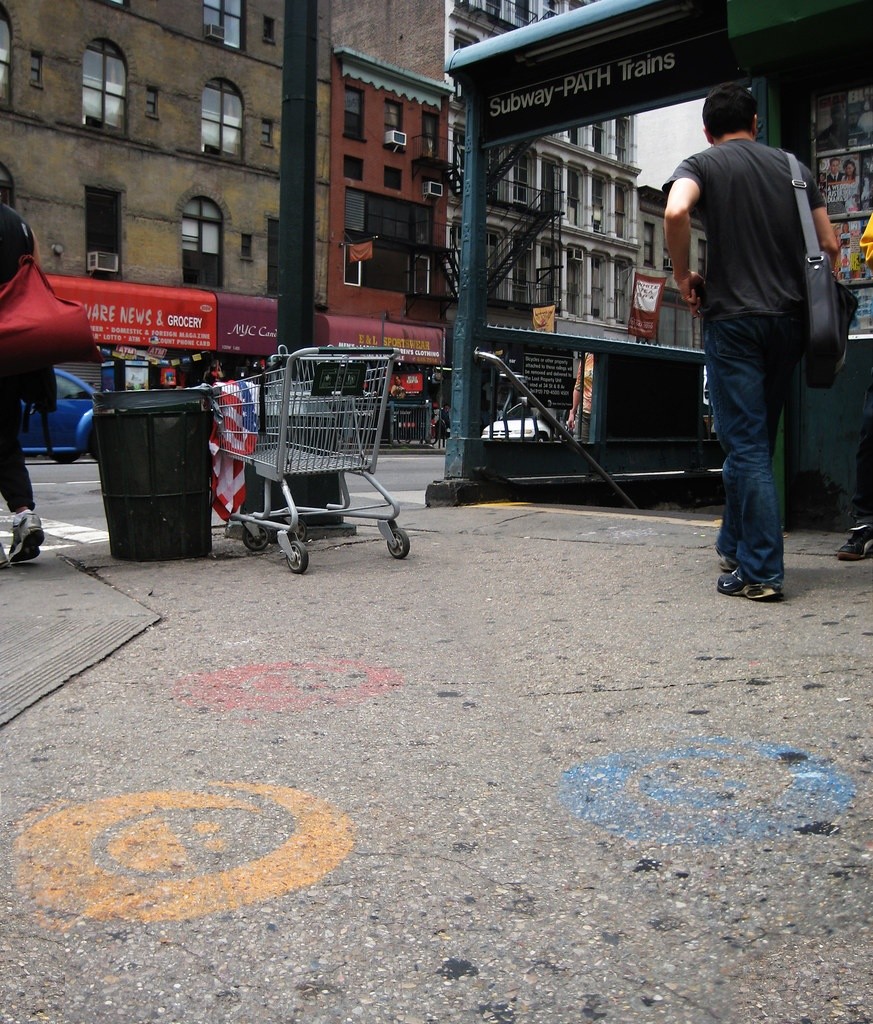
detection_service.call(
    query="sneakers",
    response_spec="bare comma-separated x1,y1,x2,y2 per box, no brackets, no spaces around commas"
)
0,544,10,568
714,541,738,572
718,567,784,599
837,522,873,560
8,509,44,561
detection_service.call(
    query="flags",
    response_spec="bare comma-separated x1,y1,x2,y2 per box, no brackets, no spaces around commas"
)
628,273,666,339
532,304,555,334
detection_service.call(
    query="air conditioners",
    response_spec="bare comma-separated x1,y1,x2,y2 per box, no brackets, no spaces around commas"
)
204,24,225,41
88,251,119,273
421,182,443,198
592,223,602,234
664,258,673,270
519,240,534,252
384,130,407,149
567,248,584,263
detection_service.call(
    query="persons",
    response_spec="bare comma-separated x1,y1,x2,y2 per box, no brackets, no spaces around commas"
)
841,221,871,278
390,376,406,399
567,352,594,442
663,83,839,602
819,158,859,213
819,99,847,151
855,94,873,139
0,200,59,568
835,208,873,560
442,406,451,438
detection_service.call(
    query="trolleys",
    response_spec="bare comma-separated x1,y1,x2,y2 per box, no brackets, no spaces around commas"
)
211,344,411,574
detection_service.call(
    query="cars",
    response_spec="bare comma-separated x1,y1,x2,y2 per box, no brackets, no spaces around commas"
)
22,368,100,464
481,403,576,442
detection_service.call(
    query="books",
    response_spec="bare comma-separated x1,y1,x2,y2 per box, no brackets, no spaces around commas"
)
815,87,873,152
859,153,873,209
818,154,861,215
841,218,873,281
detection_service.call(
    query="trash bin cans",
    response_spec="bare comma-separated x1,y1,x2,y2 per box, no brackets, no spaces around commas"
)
91,388,211,562
244,390,343,527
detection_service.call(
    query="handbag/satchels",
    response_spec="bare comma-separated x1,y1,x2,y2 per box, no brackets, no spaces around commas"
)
0,254,104,376
20,365,58,414
788,152,858,389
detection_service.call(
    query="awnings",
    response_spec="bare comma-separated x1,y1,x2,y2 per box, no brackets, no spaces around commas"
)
210,291,278,356
314,315,446,364
44,272,217,351
443,328,510,373
